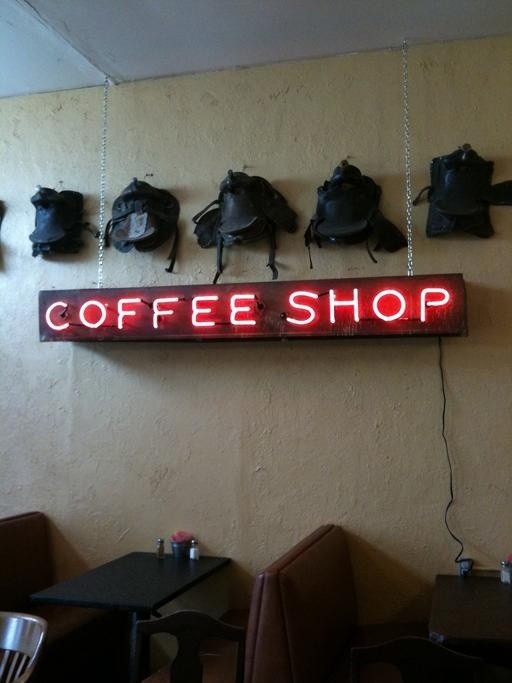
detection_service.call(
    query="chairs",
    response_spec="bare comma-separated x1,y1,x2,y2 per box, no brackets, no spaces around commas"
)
343,635,486,682
2,607,51,680
125,606,247,682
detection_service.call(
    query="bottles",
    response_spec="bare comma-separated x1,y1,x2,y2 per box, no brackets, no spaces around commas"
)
156,539,163,559
189,540,200,561
500,561,512,585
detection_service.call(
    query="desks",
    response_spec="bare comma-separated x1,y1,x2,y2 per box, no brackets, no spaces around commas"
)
425,568,511,683
30,548,232,681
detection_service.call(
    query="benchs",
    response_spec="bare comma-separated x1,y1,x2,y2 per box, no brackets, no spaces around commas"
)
141,521,425,681
1,509,116,683
